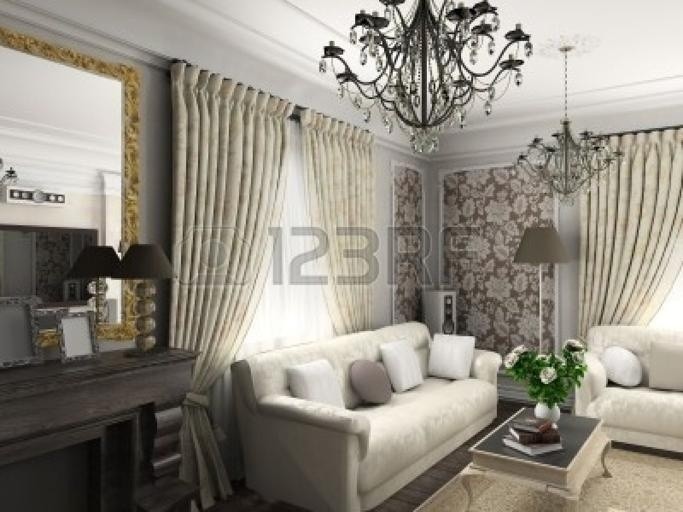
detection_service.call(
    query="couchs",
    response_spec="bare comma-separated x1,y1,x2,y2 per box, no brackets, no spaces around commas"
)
577,325,683,455
229,322,503,511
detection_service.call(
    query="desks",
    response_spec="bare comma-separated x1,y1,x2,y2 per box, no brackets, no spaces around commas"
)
0,345,201,512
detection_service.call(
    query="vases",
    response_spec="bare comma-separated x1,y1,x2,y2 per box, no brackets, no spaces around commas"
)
533,397,561,422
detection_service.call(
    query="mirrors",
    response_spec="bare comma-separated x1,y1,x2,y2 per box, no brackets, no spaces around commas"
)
0,28,140,351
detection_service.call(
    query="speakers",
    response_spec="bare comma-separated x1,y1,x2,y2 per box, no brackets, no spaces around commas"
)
422,289,456,341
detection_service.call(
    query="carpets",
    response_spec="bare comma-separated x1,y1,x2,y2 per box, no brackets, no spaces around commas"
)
415,445,682,511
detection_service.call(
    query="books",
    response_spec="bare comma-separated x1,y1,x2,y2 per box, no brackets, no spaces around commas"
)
502,423,566,458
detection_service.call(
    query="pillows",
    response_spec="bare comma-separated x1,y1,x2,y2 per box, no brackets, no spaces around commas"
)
349,360,391,403
380,338,423,394
601,345,641,387
287,358,344,410
649,340,683,389
427,334,475,380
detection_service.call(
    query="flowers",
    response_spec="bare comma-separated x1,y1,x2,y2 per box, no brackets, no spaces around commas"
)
503,339,589,409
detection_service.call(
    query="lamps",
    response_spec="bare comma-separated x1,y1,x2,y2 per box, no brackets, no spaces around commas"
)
517,43,623,202
113,244,179,353
512,225,569,354
318,0,532,154
60,244,122,325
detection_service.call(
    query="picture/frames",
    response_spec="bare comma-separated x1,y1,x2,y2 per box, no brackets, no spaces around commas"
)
54,311,98,362
0,296,45,370
